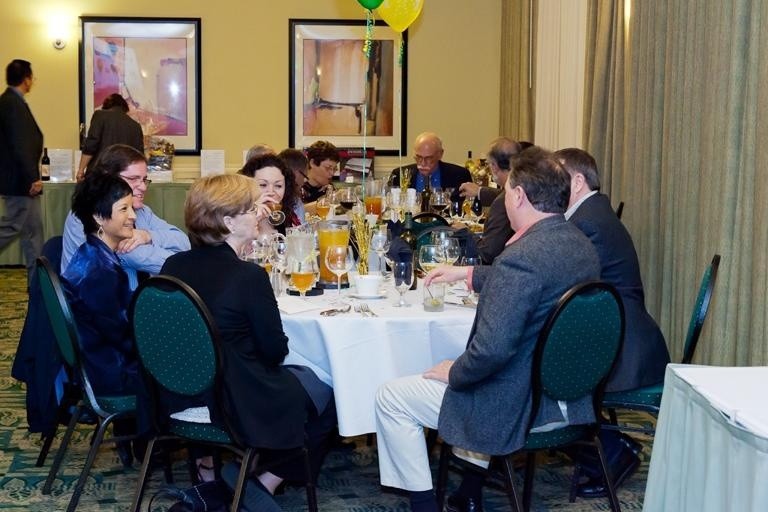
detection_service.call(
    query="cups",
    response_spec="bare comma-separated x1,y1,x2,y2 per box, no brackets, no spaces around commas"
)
316,170,448,225
317,218,351,288
421,282,446,312
355,276,380,294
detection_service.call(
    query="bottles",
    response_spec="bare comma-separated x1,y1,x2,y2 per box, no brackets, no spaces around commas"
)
40,147,51,180
400,209,419,249
450,150,492,232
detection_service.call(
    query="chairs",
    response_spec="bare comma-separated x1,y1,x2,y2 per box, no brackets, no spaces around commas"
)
128,275,319,512
35,255,137,512
603,254,721,426
433,281,626,512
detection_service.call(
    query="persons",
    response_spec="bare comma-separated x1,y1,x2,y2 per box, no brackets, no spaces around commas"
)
0,60,46,292
159,173,337,510
62,145,192,465
76,93,144,181
375,147,601,511
385,132,474,215
236,141,341,239
454,138,544,266
556,148,646,498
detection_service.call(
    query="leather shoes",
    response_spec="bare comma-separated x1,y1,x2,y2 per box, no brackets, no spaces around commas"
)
575,441,641,499
585,433,643,478
446,480,482,512
113,418,134,467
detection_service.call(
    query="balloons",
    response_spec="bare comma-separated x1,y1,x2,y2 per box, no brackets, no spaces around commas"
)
357,0,384,12
376,0,424,34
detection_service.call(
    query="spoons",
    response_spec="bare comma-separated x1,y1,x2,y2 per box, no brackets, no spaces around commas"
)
320,304,351,316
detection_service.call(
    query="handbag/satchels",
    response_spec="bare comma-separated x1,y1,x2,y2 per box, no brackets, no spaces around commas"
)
148,463,230,512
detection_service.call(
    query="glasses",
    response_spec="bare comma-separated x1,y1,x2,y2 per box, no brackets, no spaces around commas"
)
413,156,434,164
324,165,338,174
114,174,151,189
298,170,309,182
485,159,496,168
234,204,258,216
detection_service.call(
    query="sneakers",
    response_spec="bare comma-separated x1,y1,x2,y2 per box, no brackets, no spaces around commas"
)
220,460,284,512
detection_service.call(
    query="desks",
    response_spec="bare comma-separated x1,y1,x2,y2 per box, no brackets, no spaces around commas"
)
641,363,767,512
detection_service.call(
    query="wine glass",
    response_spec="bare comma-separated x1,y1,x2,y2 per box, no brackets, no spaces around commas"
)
325,244,356,303
391,263,415,306
419,230,483,283
245,227,320,299
368,227,393,280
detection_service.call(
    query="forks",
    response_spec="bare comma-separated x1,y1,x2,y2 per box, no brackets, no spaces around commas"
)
353,303,377,317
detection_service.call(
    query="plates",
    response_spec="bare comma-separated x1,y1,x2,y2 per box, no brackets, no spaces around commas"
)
349,290,387,301
445,286,476,308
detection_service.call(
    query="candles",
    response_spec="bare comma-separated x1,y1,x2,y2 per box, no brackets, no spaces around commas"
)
78,15,202,155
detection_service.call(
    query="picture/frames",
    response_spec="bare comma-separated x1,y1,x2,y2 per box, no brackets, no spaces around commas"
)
288,18,408,156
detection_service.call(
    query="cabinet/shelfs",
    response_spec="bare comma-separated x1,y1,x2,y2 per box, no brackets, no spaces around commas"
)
1,171,194,267
